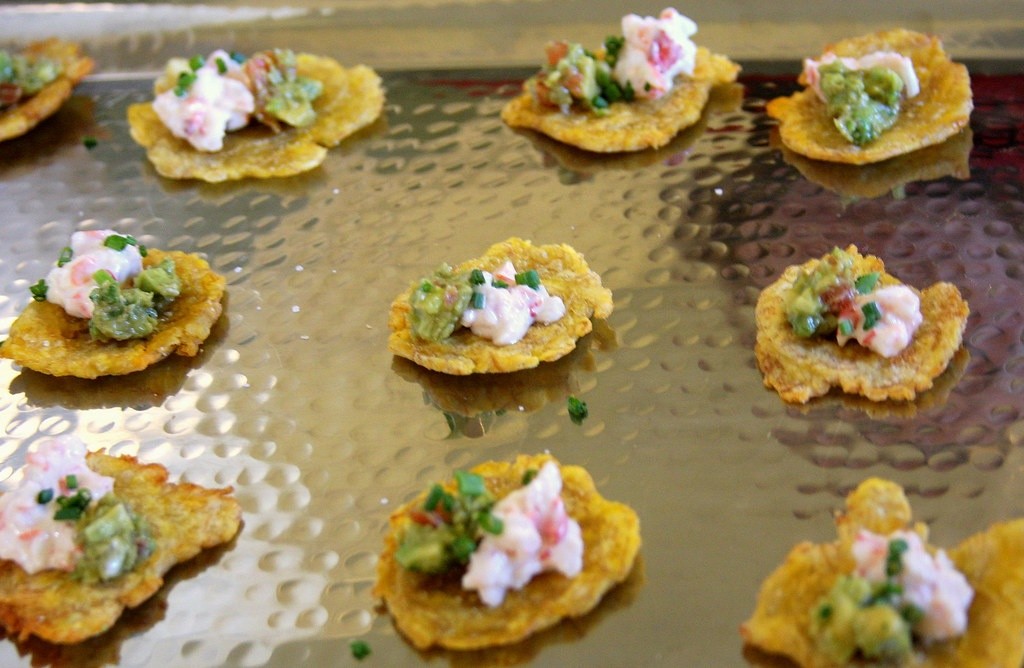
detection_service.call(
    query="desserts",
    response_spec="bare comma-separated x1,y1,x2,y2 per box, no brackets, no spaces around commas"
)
0,7,1024,668
